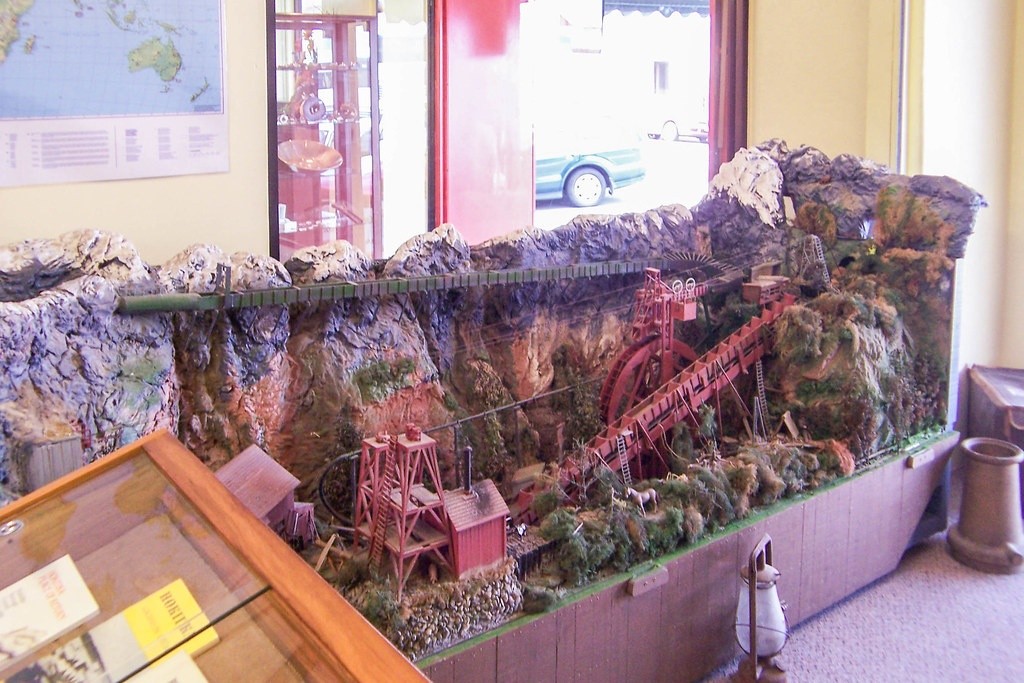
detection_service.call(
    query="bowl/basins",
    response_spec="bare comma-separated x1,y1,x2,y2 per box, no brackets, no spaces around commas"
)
278,138,343,174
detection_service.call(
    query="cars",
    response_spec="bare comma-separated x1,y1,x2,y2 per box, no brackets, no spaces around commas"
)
533,144,644,207
647,93,709,142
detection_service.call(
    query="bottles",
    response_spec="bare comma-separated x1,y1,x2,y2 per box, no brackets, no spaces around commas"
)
338,102,357,122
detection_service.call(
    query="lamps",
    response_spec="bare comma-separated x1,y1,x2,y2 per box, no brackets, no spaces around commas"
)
727,532,790,683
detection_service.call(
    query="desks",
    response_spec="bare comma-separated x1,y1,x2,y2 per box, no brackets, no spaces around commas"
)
0,429,433,683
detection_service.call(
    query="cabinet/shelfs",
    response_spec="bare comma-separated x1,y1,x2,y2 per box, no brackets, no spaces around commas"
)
274,12,374,235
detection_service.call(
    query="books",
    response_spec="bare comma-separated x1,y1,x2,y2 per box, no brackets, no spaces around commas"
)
0,554,101,671
0,577,221,683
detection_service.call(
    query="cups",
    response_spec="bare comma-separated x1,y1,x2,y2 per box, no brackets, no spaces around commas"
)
292,51,304,64
